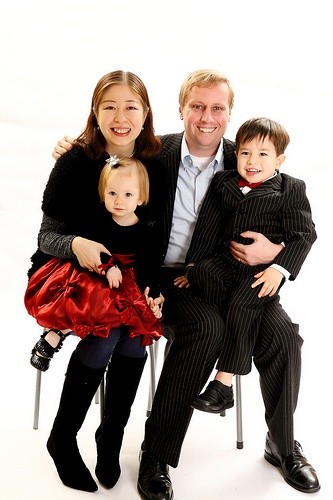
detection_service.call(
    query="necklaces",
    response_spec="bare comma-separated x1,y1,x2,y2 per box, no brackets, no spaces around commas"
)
127,151,136,158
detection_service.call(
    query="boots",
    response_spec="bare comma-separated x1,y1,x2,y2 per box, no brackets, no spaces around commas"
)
47,350,99,492
95,350,148,490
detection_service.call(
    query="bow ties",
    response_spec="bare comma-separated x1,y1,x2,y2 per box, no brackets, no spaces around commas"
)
238,179,263,189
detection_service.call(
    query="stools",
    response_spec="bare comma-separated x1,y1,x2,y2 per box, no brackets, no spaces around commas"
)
32,323,247,451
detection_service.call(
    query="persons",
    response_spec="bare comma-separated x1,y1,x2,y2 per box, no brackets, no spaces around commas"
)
29,154,166,373
28,70,173,494
52,71,321,500
175,118,317,414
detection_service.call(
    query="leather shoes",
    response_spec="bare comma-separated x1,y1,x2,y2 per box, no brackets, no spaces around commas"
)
264,432,320,494
31,346,51,371
136,440,174,500
190,381,234,413
36,328,71,357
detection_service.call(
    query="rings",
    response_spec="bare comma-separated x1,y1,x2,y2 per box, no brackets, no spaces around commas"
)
240,258,242,263
88,269,93,272
